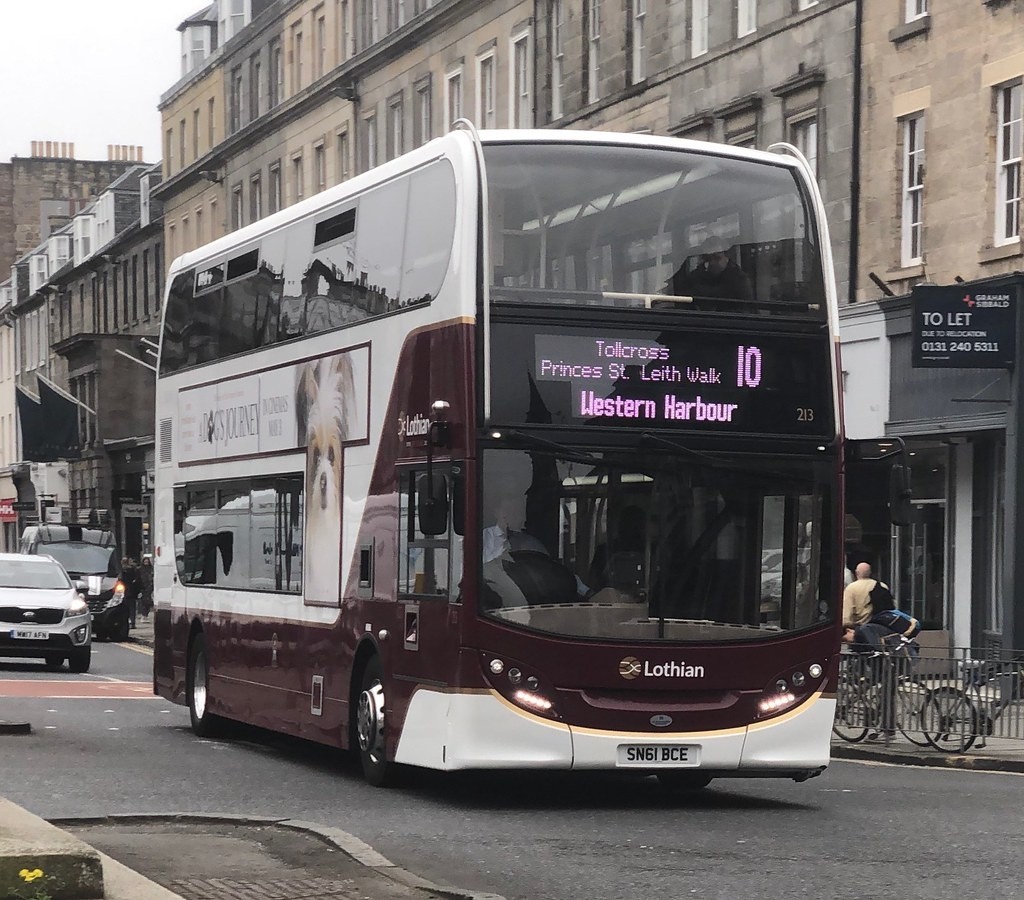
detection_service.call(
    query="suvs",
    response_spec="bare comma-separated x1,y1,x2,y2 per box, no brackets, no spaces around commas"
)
0,552,92,674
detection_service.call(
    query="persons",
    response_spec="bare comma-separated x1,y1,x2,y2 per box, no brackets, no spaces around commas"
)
840,625,920,745
842,562,890,646
479,492,595,609
681,235,756,313
790,522,852,630
117,555,153,630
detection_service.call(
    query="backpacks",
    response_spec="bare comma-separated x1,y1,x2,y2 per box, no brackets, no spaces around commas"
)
866,609,919,641
865,581,895,615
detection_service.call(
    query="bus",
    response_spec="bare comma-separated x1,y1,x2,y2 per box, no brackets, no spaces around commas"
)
150,114,843,786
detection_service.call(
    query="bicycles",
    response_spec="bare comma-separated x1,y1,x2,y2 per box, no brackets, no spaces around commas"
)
918,657,1023,753
832,634,945,748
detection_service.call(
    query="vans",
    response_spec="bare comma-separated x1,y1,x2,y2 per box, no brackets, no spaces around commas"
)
21,524,129,643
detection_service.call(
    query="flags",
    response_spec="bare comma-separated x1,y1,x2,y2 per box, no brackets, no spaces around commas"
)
15,389,59,462
39,378,83,460
